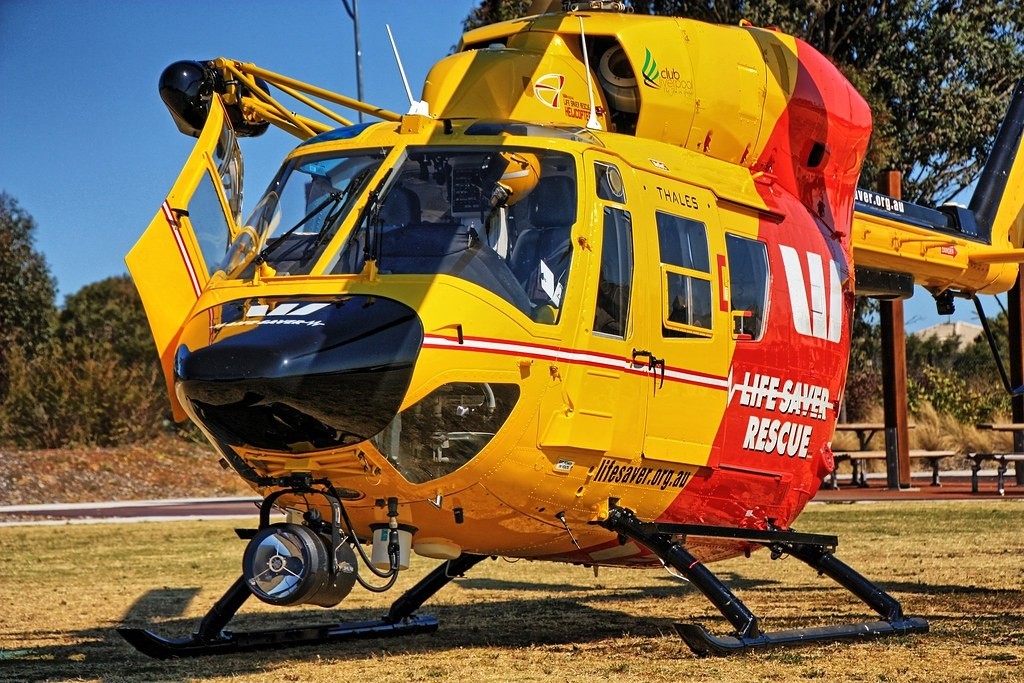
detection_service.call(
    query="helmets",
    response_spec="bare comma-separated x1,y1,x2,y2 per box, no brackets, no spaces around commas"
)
481,150,541,207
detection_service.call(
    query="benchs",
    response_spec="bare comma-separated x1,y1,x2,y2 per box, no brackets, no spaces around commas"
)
829,449,956,491
966,453,1024,496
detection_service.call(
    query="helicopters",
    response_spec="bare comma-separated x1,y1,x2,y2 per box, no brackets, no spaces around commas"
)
116,1,1023,663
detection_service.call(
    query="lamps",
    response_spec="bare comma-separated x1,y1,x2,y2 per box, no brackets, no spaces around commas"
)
242,486,359,609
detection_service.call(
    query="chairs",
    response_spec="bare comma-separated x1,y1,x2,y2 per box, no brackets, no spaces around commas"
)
510,176,578,310
359,184,422,229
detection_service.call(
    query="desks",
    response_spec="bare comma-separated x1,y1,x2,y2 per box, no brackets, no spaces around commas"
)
835,422,917,488
976,422,1024,432
